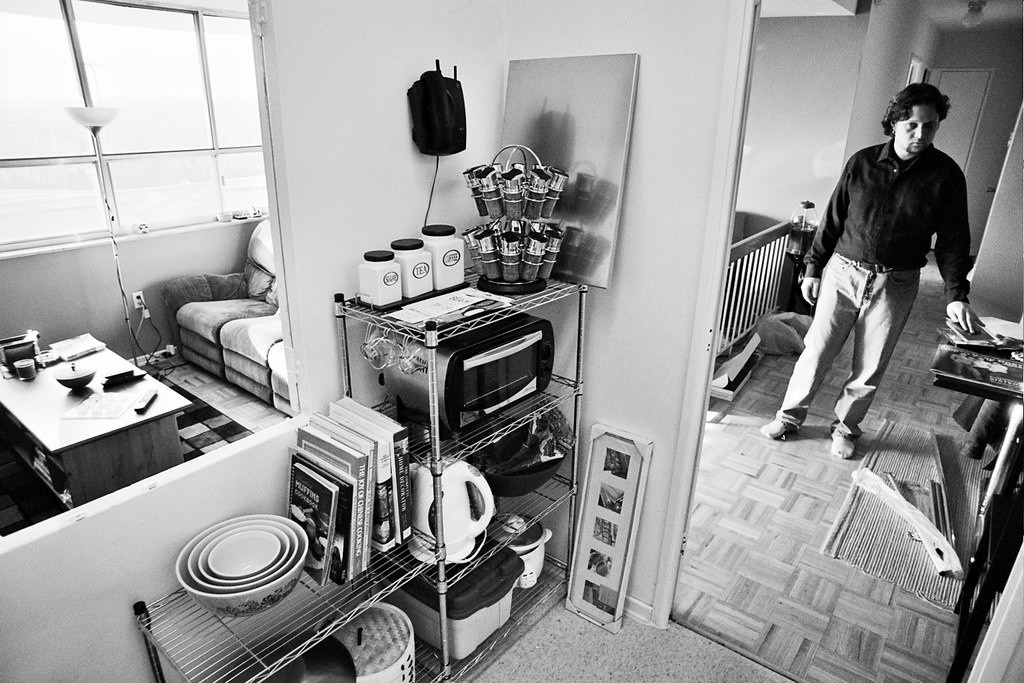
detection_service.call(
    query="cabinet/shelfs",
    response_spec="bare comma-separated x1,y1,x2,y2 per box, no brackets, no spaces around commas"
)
133,284,579,682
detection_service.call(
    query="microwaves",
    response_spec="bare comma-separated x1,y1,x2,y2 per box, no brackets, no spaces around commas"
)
381,311,554,439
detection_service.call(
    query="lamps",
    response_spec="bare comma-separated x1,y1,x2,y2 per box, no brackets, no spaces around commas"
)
961,0,987,28
68,108,139,368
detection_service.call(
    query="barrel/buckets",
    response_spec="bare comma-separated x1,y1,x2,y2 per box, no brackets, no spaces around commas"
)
313,600,417,682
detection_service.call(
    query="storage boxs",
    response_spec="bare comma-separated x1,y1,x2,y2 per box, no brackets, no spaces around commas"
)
373,537,524,658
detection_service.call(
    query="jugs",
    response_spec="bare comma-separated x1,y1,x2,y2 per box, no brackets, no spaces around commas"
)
791,200,819,233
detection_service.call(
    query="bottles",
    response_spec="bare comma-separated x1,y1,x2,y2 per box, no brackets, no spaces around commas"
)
419,225,465,291
391,239,433,299
358,250,403,306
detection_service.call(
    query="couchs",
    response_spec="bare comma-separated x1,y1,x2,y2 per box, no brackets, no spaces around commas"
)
165,220,291,416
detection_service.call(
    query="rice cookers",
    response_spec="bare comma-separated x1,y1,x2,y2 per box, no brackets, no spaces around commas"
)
487,511,552,590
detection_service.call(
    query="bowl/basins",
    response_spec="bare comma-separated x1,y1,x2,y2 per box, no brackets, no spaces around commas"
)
465,451,568,498
56,369,97,390
223,630,356,683
174,514,313,616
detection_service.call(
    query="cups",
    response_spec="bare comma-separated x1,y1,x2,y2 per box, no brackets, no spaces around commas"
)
554,174,621,276
14,359,36,381
464,162,568,283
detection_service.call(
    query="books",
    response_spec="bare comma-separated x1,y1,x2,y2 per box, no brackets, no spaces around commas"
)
285,394,414,587
49,332,107,362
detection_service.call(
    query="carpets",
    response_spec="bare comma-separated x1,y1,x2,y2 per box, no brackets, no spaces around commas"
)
823,416,996,613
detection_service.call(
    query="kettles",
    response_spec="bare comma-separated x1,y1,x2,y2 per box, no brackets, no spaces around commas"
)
408,456,495,565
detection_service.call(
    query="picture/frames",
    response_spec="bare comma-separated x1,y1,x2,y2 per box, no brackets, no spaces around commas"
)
566,423,653,634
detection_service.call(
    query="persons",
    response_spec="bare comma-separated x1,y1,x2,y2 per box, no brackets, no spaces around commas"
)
759,80,986,460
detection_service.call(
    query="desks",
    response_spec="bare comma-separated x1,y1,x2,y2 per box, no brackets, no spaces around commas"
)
0,334,194,510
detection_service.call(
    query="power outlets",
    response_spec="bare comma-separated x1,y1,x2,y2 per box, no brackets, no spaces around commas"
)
133,292,144,308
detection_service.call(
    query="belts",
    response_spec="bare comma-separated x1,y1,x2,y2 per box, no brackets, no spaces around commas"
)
860,262,890,272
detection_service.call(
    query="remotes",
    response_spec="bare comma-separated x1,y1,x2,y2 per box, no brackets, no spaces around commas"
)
134,388,158,413
100,370,146,387
